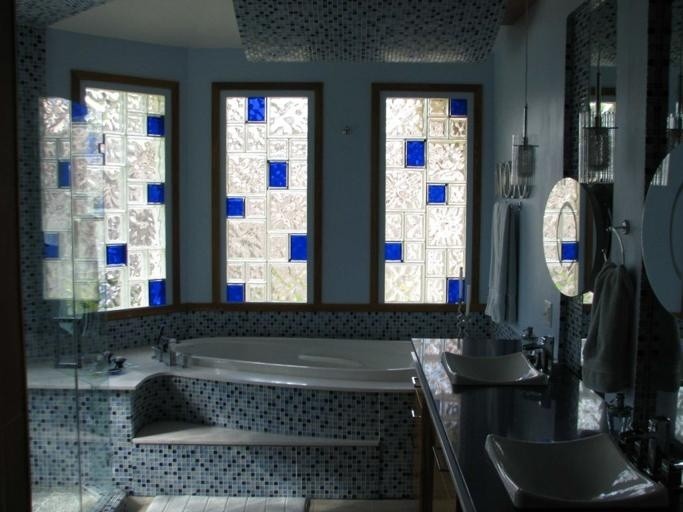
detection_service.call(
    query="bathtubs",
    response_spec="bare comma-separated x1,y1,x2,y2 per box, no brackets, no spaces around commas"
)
163,332,420,395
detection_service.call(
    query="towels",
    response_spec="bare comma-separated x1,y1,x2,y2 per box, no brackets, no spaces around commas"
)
484,200,519,325
580,261,635,395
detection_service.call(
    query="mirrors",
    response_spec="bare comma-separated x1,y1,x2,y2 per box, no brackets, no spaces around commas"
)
558,3,618,389
637,1,682,444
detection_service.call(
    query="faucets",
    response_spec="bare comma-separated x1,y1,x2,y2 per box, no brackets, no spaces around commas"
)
522,335,555,367
618,415,672,482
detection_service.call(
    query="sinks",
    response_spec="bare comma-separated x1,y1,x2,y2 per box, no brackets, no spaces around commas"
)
440,348,545,388
483,429,666,509
